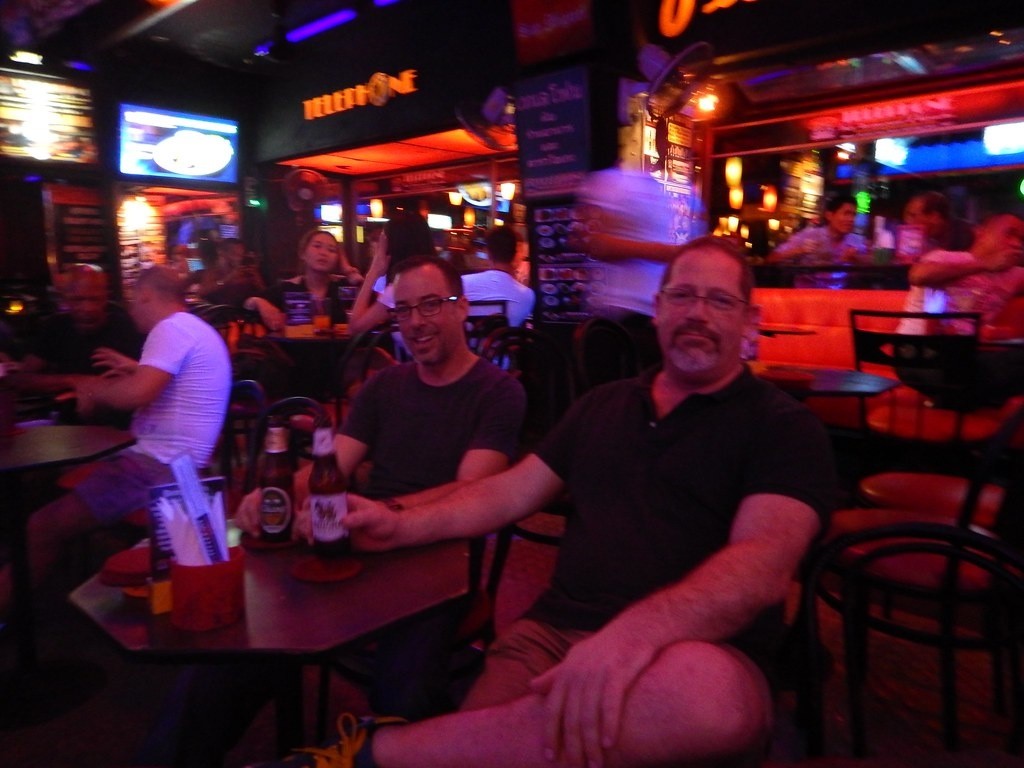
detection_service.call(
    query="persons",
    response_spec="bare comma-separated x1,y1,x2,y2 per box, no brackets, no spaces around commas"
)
30,265,229,591
768,194,871,265
0,264,142,426
349,209,434,361
237,230,362,331
197,239,265,302
134,254,525,768
577,164,709,377
248,235,831,768
893,192,962,264
898,211,1024,336
461,227,535,371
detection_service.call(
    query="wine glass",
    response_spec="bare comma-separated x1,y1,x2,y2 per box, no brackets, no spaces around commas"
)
338,286,357,322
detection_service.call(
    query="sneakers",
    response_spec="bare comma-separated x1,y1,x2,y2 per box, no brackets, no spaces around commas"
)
247,711,411,768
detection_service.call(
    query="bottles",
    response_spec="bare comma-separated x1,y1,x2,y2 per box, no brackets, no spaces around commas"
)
308,415,350,560
258,416,294,544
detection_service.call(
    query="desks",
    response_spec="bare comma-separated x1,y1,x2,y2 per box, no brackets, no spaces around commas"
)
67,516,472,764
741,364,895,414
0,425,138,508
236,318,351,376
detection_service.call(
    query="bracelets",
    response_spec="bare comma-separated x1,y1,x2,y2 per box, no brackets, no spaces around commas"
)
383,497,401,510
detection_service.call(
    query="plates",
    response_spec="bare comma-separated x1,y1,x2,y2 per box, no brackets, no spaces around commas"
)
151,134,234,177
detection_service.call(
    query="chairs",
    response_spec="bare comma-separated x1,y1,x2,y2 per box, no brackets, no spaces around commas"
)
464,299,553,368
786,311,1024,761
219,324,401,489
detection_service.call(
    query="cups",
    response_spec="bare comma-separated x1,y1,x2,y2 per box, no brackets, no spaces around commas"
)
171,545,247,631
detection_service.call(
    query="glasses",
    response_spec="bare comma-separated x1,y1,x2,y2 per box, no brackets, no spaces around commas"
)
387,295,461,324
658,287,748,312
